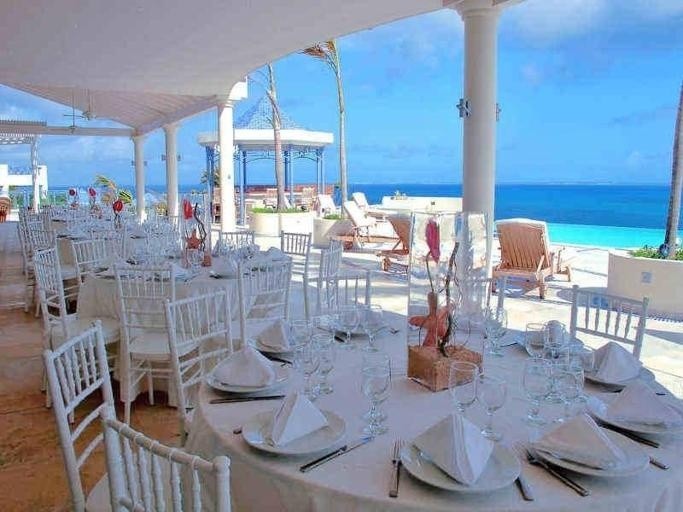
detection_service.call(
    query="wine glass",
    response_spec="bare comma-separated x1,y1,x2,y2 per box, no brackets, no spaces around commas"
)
475,373,508,444
186,248,204,274
483,307,511,359
521,344,595,425
448,361,478,415
357,304,383,352
338,305,360,351
292,319,334,397
359,346,391,437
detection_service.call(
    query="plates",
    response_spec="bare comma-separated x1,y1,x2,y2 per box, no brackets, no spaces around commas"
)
208,268,246,278
529,425,650,478
205,361,288,393
584,364,655,387
514,331,583,350
242,406,347,456
587,392,683,434
332,315,384,335
248,334,294,354
400,437,520,493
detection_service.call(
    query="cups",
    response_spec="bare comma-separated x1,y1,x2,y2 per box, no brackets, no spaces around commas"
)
525,323,547,356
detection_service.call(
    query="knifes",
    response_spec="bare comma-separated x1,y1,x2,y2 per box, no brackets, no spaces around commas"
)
208,394,285,405
298,431,372,473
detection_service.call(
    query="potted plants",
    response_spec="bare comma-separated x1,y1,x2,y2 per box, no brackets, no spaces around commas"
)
300,40,364,250
247,60,315,240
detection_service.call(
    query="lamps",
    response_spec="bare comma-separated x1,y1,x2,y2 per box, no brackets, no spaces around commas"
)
454,97,470,120
496,100,501,121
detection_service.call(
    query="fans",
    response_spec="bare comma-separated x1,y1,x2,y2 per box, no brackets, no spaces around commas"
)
50,90,93,132
61,91,124,123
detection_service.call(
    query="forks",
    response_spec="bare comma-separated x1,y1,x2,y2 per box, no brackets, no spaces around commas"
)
389,437,401,498
512,439,606,497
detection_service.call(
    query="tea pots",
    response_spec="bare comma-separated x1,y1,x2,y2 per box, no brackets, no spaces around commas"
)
543,320,565,347
